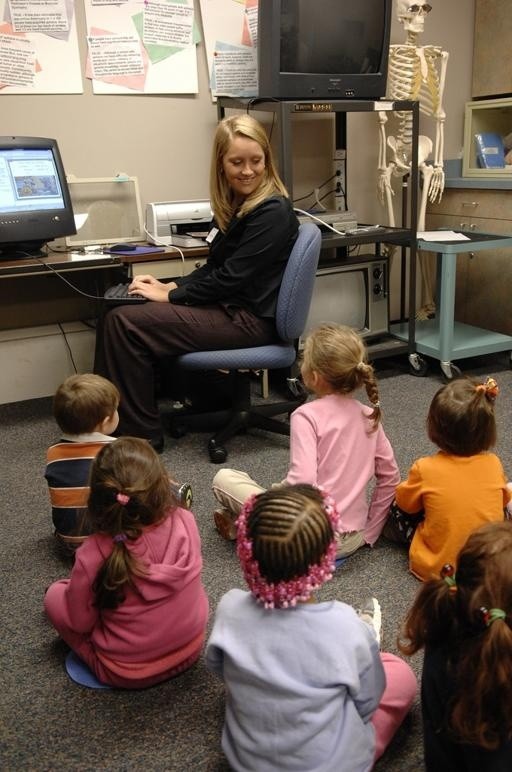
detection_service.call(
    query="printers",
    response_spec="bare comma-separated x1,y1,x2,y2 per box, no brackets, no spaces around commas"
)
146,199,215,248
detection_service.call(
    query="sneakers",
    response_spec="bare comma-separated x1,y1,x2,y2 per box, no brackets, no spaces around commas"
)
354,596,382,648
212,506,241,540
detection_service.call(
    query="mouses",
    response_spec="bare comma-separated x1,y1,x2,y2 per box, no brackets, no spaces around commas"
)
110,242,136,252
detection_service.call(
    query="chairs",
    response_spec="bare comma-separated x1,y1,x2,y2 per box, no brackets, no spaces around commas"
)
166,223,322,463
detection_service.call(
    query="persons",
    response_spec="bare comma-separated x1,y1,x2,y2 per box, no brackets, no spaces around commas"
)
211,323,401,560
397,522,512,772
205,483,417,772
384,376,512,582
45,373,120,558
95,114,301,453
44,436,209,690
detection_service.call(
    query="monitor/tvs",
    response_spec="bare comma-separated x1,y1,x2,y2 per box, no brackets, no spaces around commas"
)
257,0,392,98
0,136,77,261
297,254,390,353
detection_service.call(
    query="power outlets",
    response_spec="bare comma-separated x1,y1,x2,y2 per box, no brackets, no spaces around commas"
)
333,160,345,197
334,149,346,160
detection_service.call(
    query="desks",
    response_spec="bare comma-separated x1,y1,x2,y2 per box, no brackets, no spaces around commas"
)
0,240,270,399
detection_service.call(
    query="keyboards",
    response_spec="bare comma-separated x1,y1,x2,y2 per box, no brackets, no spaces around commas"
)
104,283,148,304
